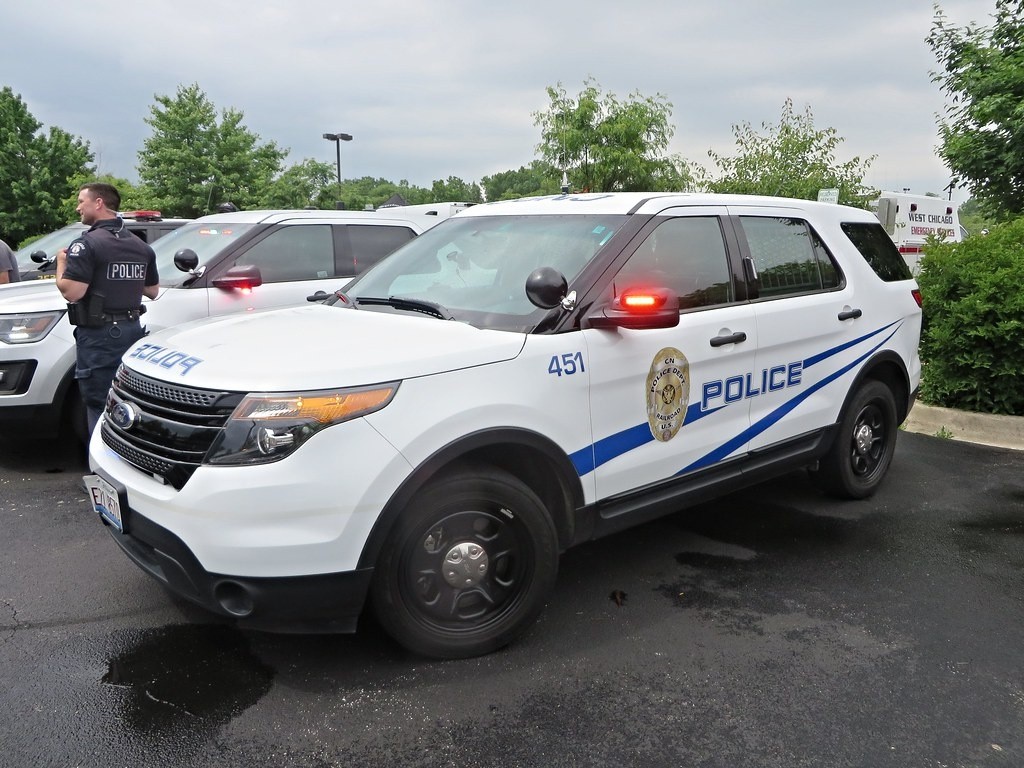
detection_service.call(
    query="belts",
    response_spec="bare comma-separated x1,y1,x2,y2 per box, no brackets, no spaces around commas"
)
104,313,130,321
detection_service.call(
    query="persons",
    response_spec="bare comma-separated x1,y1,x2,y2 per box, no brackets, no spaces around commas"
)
0,239,21,285
56,182,158,493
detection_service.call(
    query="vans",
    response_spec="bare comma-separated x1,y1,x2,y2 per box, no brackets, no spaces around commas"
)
817,187,970,286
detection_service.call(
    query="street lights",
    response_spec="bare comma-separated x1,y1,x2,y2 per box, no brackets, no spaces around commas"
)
322,132,353,210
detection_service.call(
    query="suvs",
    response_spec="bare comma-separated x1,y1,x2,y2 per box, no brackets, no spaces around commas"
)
0,209,499,457
8,210,196,282
83,191,924,661
